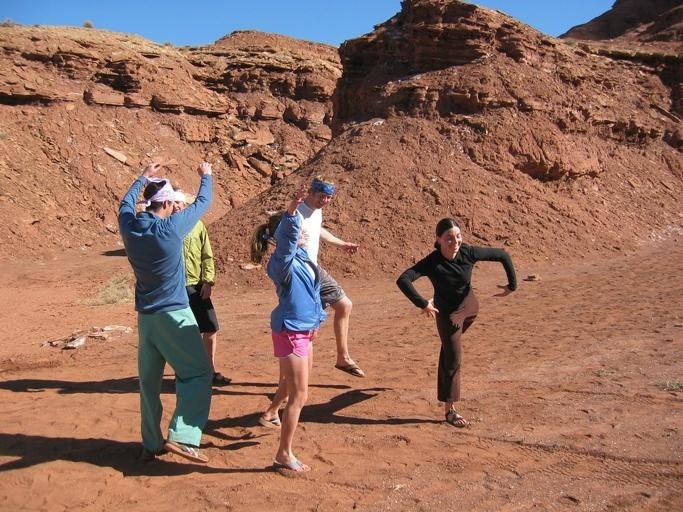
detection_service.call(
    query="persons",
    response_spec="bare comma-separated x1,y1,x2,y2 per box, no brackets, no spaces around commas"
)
117,162,215,464
394,218,518,428
166,185,232,387
247,183,320,472
275,172,368,403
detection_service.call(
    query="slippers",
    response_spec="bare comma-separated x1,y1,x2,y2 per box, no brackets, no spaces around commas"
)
333,363,365,379
444,408,467,427
257,416,281,431
272,459,310,472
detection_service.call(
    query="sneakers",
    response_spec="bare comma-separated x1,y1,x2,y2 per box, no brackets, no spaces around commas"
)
162,441,209,465
211,373,232,385
141,448,191,464
